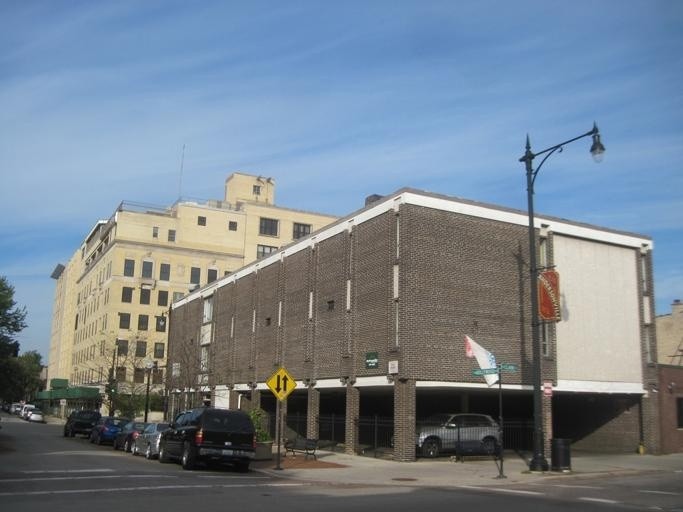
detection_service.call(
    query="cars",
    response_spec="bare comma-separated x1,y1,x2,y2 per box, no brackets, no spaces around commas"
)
391,414,503,458
5,403,43,422
64,408,256,470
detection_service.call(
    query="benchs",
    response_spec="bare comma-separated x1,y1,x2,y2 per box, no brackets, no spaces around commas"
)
284,439,318,460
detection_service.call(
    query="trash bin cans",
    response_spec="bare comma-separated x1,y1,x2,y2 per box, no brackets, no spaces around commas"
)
549,439,573,473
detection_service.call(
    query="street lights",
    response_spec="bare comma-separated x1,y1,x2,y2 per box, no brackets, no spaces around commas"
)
519,120,605,471
145,357,155,422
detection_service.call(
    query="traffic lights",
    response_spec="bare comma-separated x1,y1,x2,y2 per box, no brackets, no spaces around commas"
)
106,379,117,400
